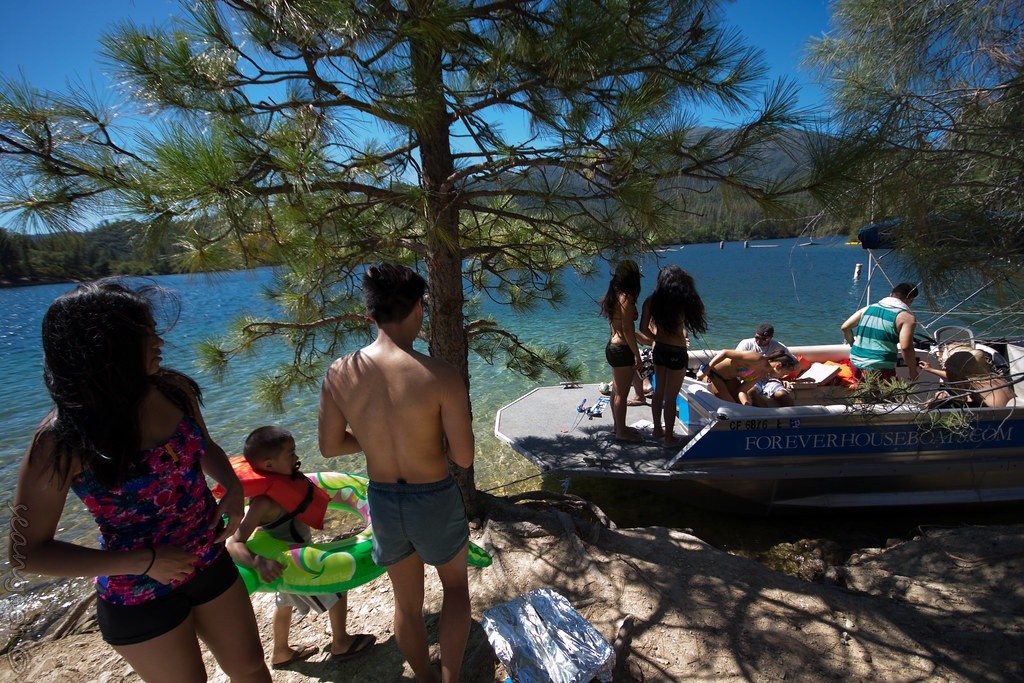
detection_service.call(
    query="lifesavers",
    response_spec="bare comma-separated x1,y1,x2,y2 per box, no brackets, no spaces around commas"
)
223,471,494,598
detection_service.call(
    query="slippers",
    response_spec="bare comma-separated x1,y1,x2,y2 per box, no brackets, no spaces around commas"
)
271,645,319,668
331,631,377,663
650,430,668,439
613,425,645,444
662,435,687,448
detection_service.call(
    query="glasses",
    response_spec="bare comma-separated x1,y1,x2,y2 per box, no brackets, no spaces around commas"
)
755,336,769,341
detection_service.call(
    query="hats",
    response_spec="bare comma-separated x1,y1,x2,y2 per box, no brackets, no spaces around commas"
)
757,323,774,337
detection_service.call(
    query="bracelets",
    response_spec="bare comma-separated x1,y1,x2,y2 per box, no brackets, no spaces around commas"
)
141,543,156,577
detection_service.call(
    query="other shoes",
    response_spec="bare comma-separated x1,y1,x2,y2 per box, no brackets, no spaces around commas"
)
625,397,646,404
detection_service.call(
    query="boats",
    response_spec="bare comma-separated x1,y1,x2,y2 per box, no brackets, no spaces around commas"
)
495,326,1024,509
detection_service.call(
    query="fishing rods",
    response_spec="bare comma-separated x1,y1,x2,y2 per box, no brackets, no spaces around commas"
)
565,273,715,366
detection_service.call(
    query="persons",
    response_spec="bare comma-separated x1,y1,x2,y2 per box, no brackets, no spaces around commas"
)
600,260,644,443
319,260,474,683
627,264,707,448
697,322,800,408
919,349,1014,409
8,279,272,683
841,282,919,405
225,425,377,668
